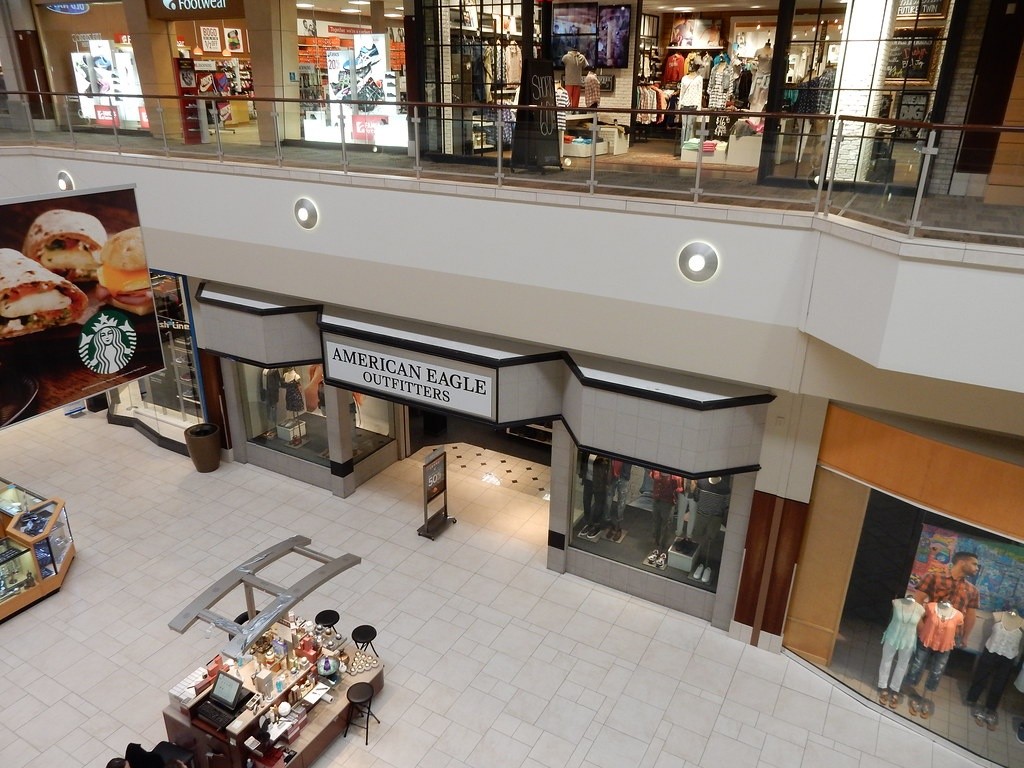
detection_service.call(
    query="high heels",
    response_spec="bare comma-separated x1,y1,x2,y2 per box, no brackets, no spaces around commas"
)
289,435,297,444
294,437,302,446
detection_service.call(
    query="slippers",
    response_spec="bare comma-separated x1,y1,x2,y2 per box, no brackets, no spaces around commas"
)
908,696,919,715
920,699,930,718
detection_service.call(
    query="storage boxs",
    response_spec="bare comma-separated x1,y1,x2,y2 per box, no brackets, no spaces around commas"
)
298,36,404,70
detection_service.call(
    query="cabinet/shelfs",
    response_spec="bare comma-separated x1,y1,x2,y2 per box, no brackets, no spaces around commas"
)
161,616,384,768
216,57,254,102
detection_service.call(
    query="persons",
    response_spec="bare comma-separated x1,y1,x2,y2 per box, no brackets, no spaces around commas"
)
106,758,130,768
554,80,571,157
302,20,317,37
386,28,405,42
578,451,631,525
795,65,836,163
316,375,359,452
914,551,981,647
749,42,773,126
259,367,304,424
674,19,693,37
698,20,720,45
967,610,1024,713
0,66,8,107
649,470,731,550
561,49,601,108
679,69,701,147
706,59,735,109
878,597,926,709
908,601,965,715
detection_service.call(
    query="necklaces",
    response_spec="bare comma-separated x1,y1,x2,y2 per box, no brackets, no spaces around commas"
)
937,608,952,632
900,600,914,624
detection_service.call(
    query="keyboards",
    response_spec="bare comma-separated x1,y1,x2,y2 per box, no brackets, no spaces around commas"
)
197,701,235,728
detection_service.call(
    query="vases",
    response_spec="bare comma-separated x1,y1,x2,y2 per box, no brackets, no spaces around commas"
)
184,422,220,473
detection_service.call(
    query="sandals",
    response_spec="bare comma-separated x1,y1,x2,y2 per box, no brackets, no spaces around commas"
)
879,687,888,704
889,691,899,708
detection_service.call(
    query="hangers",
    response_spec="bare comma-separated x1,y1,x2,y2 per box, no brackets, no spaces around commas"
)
639,84,655,90
673,49,724,58
461,34,518,46
800,65,819,82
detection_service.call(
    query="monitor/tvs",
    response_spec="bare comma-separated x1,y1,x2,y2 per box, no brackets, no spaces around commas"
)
209,670,243,710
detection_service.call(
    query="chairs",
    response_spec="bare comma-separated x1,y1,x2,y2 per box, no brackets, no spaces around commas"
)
126,741,196,768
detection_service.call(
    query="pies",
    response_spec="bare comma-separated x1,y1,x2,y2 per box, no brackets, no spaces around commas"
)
0,247,90,340
22,208,107,291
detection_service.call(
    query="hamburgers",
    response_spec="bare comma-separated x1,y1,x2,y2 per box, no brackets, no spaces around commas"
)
95,226,154,316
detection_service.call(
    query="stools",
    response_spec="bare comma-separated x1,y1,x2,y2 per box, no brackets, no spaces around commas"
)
315,610,340,634
229,610,261,640
351,625,379,658
343,682,380,744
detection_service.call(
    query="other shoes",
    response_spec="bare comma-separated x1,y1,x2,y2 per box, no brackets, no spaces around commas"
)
606,528,616,538
976,711,986,726
1016,722,1024,744
986,712,998,731
682,543,694,554
676,537,689,551
613,531,622,541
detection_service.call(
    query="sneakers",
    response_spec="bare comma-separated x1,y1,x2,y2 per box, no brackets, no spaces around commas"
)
175,336,192,345
648,549,658,564
693,564,703,579
343,44,381,71
341,77,385,112
83,54,117,71
586,527,601,538
182,387,198,397
176,354,192,363
580,525,594,535
702,567,711,582
180,372,196,381
655,553,666,568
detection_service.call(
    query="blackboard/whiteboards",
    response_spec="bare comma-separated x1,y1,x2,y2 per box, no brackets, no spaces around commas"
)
510,58,565,170
